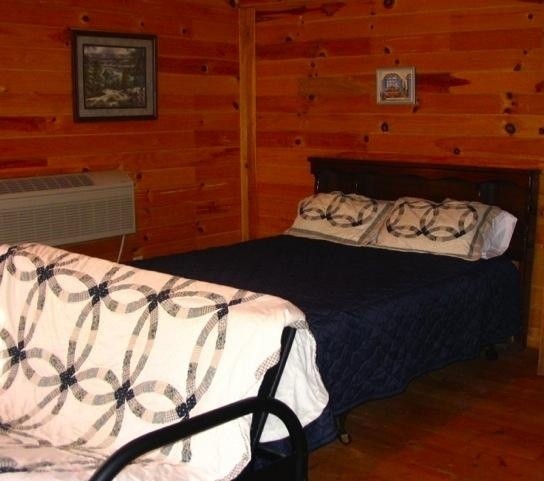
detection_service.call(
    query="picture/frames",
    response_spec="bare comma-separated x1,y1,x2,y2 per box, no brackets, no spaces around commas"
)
375,66,417,107
70,29,158,122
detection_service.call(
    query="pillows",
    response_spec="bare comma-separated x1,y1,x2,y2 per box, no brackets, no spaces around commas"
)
284,190,520,262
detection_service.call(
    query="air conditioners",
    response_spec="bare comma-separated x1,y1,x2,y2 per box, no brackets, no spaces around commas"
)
0,170,139,248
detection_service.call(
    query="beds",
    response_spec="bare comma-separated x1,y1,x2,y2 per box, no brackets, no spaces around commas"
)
127,157,543,475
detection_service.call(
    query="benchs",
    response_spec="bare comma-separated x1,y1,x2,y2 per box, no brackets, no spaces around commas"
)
0,243,310,481
